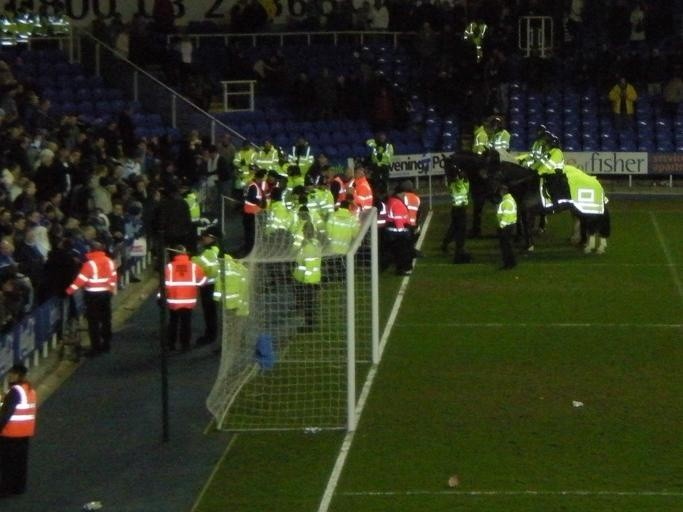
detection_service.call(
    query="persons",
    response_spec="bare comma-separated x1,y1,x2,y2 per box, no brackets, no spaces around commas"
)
401,1,682,130
0,363,36,498
1,1,423,357
441,116,610,267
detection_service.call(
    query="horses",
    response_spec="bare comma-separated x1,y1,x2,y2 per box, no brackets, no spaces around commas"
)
441,150,548,239
476,146,611,256
